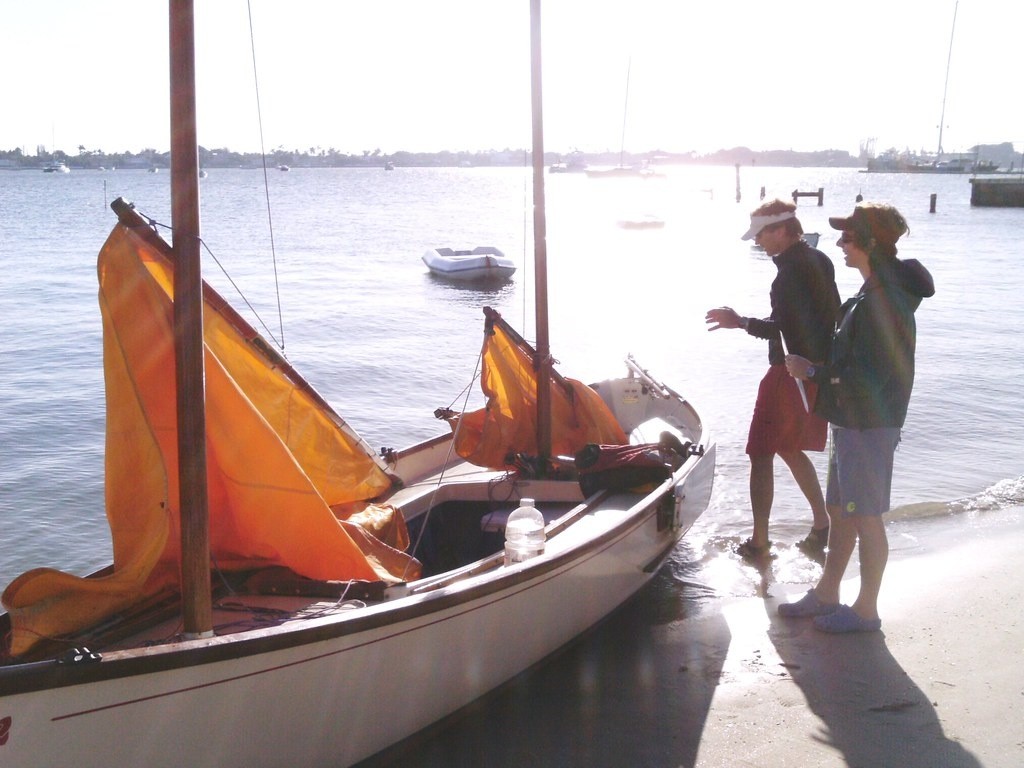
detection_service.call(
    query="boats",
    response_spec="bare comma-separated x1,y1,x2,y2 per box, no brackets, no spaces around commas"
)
43,159,70,173
385,161,394,170
277,165,290,172
97,166,115,172
547,162,664,178
198,170,207,178
148,167,158,173
421,246,517,278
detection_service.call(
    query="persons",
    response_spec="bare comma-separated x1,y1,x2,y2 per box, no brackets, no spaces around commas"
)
705,201,841,560
777,205,936,633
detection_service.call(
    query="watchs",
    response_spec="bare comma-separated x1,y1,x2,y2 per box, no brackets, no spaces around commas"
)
806,364,816,380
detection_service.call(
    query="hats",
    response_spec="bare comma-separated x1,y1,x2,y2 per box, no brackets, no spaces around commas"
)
828,203,899,247
741,210,797,241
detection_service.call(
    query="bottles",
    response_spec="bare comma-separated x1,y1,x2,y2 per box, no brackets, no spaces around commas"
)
504,498,545,567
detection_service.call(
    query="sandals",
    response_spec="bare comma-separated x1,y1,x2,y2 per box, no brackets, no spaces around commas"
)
733,537,772,559
804,526,830,547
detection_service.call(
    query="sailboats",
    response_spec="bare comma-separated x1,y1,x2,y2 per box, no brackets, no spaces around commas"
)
859,0,1001,174
0,0,717,768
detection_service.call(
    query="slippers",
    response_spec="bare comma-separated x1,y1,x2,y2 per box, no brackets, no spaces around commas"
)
777,588,882,633
